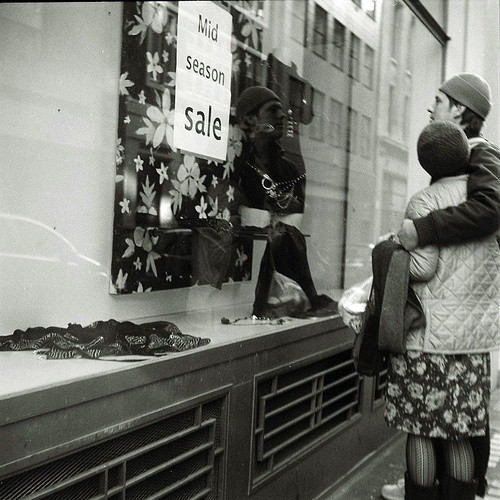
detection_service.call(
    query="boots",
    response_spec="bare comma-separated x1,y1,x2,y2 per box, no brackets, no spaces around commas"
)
404,470,440,500
436,472,476,500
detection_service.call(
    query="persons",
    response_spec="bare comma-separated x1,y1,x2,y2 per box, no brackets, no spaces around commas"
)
170,84,305,285
382,121,500,500
383,71,500,500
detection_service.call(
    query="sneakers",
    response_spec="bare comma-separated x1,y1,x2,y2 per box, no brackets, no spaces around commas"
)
382,478,405,500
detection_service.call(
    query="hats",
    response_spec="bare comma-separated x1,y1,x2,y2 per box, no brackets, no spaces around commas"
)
236,86,281,123
439,73,492,121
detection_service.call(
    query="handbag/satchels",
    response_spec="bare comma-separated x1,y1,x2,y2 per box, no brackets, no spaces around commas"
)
354,300,392,378
